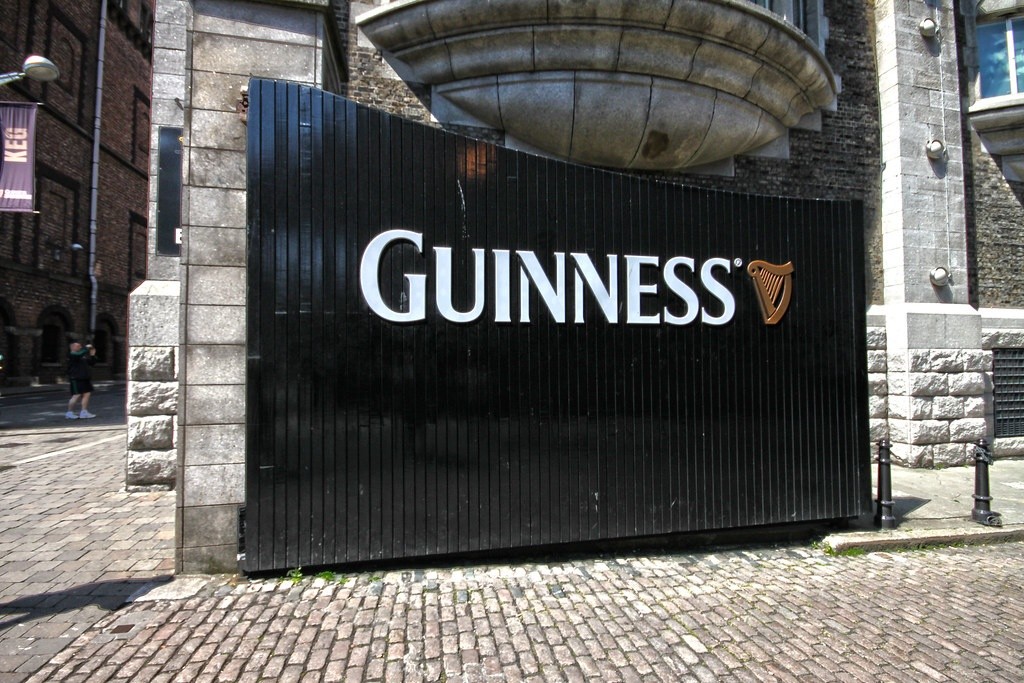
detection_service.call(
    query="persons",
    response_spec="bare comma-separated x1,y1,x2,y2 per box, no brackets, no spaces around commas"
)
66,338,97,420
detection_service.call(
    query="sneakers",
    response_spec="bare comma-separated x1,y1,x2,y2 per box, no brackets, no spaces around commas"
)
66,411,78,419
79,410,96,418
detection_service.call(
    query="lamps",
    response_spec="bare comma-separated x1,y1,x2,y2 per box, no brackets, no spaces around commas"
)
0,55,60,88
54,243,85,261
